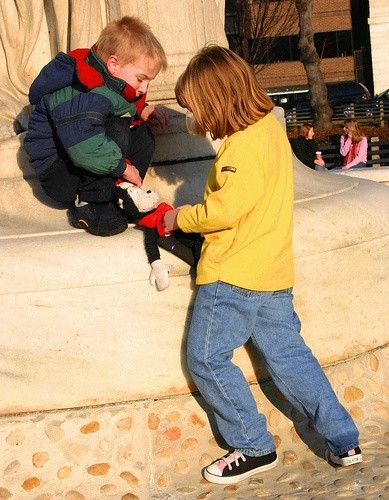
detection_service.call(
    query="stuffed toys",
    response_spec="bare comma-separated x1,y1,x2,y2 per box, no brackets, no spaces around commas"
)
113,159,206,292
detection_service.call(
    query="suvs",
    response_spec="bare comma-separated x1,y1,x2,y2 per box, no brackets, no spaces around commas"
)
283,81,372,124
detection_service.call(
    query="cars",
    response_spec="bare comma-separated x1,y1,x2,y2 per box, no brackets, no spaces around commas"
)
355,89,389,119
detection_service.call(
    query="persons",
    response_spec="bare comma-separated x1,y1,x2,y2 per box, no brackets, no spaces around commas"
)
23,16,168,237
336,118,368,170
292,124,326,171
163,41,363,485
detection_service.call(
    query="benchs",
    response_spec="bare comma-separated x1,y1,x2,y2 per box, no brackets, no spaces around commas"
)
314,141,389,168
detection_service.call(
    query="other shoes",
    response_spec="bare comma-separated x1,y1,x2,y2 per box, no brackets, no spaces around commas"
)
71,197,129,237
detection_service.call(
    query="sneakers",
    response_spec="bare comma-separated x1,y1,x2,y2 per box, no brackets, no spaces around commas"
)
202,450,280,484
324,444,363,467
